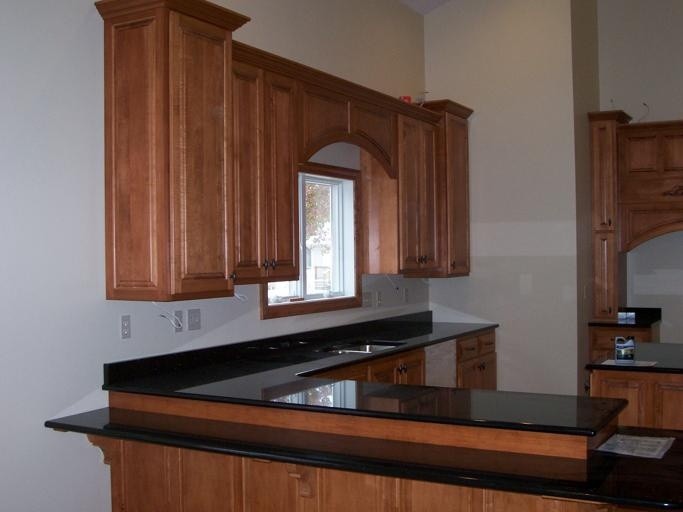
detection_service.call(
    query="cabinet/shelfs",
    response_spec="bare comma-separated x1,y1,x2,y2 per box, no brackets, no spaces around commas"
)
457,330,497,390
589,369,683,431
617,127,682,212
232,58,300,283
366,351,425,386
102,10,236,303
589,326,649,350
359,114,439,277
441,113,469,277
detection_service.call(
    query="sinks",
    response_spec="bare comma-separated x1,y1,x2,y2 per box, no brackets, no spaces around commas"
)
351,340,407,353
324,346,367,356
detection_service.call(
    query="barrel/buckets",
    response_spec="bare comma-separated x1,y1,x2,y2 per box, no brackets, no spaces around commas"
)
614,335,635,364
